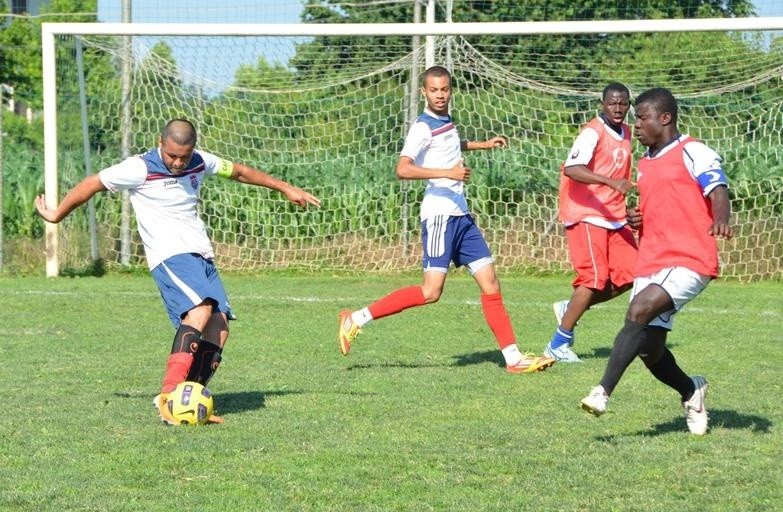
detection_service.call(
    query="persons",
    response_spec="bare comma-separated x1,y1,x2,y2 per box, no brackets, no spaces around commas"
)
330,64,557,375
571,86,736,438
31,118,322,426
538,81,643,365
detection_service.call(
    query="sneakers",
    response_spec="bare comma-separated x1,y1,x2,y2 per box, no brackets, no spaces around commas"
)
542,340,583,362
506,356,555,372
154,393,224,425
681,375,711,435
579,385,609,418
336,310,362,356
553,299,574,347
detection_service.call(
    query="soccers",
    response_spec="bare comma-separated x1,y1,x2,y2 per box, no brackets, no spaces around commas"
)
166,380,213,425
